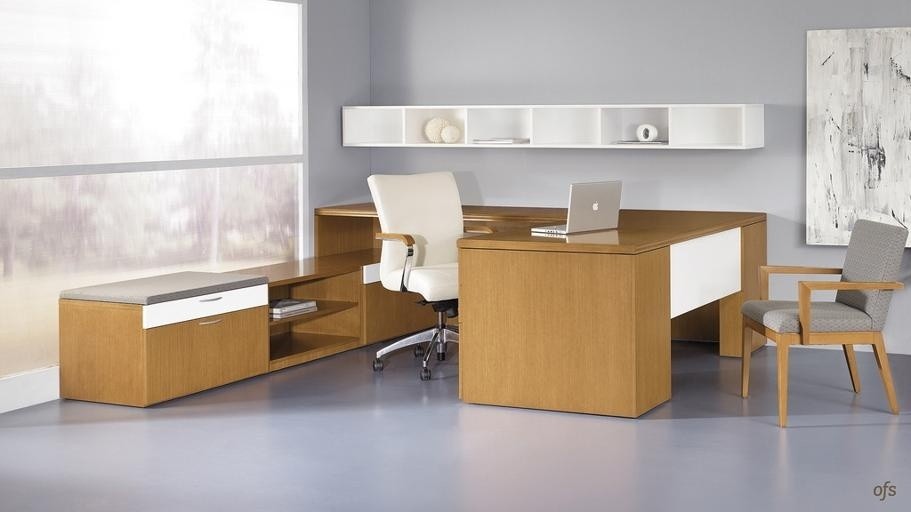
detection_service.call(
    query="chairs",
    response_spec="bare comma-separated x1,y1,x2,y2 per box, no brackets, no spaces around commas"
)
367,170,465,379
740,219,909,427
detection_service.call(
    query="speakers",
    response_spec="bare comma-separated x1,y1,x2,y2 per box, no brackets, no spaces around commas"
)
635,124,658,141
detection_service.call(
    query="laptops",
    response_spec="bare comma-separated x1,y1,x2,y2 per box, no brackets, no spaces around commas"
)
530,180,622,236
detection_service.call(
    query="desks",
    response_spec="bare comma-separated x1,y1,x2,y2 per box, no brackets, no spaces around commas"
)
314,181,767,418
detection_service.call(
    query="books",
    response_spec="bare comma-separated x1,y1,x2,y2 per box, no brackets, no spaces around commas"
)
268,297,317,319
472,136,529,145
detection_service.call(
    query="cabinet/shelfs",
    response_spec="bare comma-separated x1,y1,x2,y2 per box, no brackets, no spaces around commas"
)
58,270,269,409
342,104,764,150
222,259,366,374
322,246,449,345
